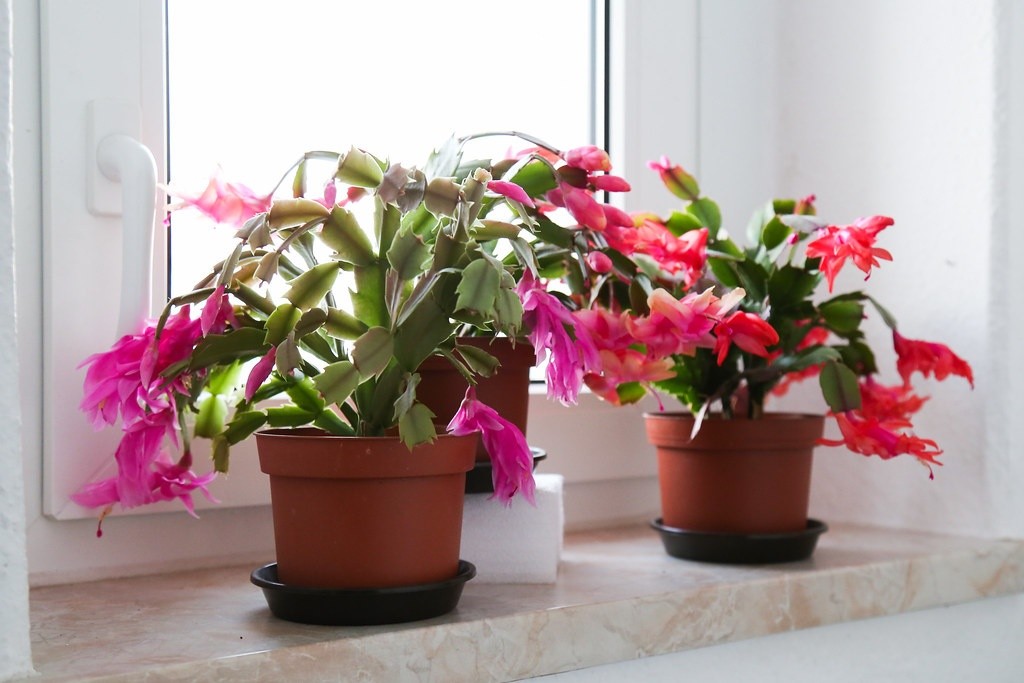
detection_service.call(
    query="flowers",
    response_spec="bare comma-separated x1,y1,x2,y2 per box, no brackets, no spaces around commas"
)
571,162,975,477
67,132,600,518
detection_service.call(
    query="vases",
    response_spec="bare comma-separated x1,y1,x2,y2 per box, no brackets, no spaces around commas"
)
642,409,825,533
254,426,480,587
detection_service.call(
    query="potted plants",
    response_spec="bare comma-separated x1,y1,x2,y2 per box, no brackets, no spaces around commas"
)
297,132,565,460
